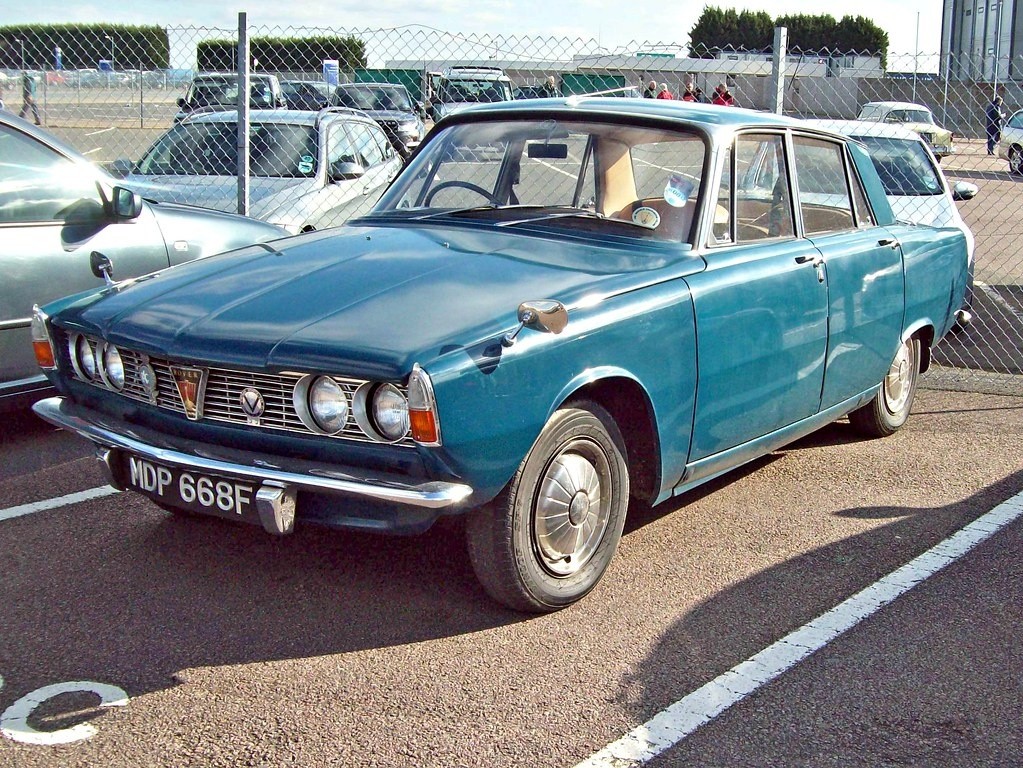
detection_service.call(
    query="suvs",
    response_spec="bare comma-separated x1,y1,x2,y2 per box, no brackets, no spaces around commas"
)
419,64,514,127
325,80,426,163
107,104,410,242
171,72,290,127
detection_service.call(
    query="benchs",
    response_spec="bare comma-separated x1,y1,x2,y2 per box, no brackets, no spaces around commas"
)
715,198,853,242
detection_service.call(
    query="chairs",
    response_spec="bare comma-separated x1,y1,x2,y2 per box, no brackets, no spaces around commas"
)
620,198,729,246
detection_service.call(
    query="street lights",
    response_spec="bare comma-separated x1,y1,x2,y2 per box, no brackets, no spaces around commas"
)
15,40,25,71
105,35,114,70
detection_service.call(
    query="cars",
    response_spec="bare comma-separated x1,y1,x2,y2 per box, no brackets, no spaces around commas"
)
855,101,954,163
62,90,971,617
262,80,338,113
997,108,1023,176
0,107,293,416
512,85,549,101
0,67,195,94
740,118,981,332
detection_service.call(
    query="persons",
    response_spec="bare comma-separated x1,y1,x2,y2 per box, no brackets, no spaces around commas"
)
536,76,558,98
18,70,43,125
644,81,733,106
986,95,1006,155
